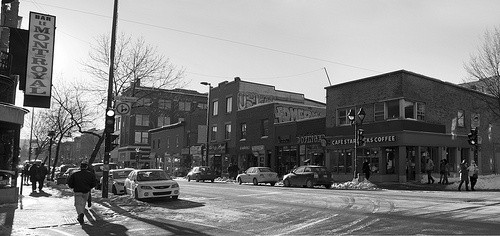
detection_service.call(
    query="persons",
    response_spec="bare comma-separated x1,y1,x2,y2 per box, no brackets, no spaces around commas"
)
38,163,47,191
438,159,450,184
228,163,240,180
426,157,434,184
29,163,38,192
364,162,371,180
86,163,96,207
467,160,478,190
24,164,31,186
68,162,98,224
457,161,469,191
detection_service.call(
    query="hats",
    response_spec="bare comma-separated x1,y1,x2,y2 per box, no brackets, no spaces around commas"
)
80,162,88,167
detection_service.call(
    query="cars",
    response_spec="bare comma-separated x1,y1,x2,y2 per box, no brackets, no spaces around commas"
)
91,162,120,182
49,164,80,182
100,168,136,194
282,164,333,188
124,169,180,200
187,166,216,182
237,166,279,186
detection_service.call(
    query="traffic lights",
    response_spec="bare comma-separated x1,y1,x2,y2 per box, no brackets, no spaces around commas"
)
110,134,120,152
357,129,365,146
106,107,115,133
467,129,476,145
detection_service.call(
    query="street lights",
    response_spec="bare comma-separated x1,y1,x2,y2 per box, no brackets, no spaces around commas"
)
200,81,212,165
348,107,366,181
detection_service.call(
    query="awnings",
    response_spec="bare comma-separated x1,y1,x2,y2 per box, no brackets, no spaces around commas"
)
303,159,310,163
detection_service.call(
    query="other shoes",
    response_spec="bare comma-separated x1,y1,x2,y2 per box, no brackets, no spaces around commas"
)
427,182,431,184
458,186,461,191
432,180,434,183
78,213,84,223
